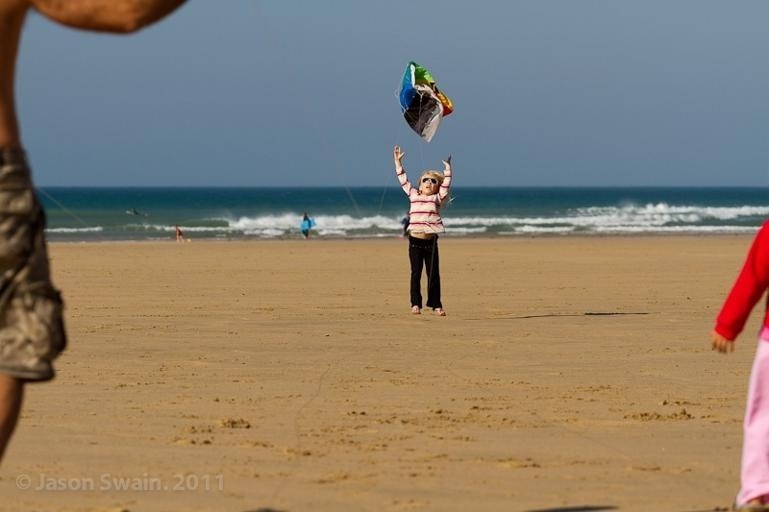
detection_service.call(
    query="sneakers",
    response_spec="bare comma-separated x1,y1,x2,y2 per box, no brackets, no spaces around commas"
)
435,308,445,316
412,306,420,314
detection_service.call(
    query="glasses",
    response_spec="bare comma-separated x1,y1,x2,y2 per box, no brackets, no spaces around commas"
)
422,178,437,184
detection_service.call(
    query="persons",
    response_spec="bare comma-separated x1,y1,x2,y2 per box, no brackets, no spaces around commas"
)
175,223,184,242
131,207,138,215
1,1,190,461
393,144,451,317
399,211,411,237
708,218,769,511
301,213,311,240
